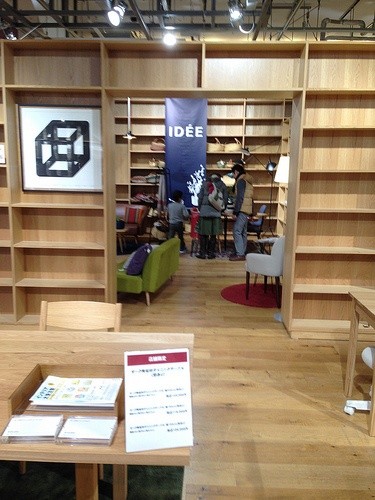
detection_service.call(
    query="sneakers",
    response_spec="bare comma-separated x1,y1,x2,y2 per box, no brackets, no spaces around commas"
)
229,254,244,261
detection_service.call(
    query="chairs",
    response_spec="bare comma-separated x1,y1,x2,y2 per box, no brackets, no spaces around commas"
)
244,236,285,299
247,205,266,239
18,300,122,480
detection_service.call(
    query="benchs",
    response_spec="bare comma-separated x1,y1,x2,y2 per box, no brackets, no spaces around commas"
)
116,203,146,244
116,238,181,306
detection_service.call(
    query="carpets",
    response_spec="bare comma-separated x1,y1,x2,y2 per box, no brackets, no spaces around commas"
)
191,240,268,259
222,283,283,308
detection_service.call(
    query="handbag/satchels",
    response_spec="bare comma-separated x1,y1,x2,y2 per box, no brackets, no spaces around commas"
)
207,187,225,212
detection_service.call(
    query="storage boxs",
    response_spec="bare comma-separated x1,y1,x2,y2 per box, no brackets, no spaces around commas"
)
9,364,124,423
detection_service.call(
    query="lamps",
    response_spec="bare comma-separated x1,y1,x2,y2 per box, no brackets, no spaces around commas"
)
107,0,127,27
240,138,279,156
267,159,277,172
242,154,275,236
227,0,244,20
122,131,137,139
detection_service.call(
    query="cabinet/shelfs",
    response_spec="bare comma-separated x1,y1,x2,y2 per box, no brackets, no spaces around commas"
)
0,39,375,337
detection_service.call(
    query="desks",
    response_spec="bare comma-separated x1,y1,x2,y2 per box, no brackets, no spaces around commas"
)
190,208,259,250
0,330,194,500
345,291,375,436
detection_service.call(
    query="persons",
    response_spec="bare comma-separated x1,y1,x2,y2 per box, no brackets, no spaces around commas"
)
196,173,226,260
229,165,254,261
167,194,189,253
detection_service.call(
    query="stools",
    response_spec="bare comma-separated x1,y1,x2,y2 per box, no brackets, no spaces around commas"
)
344,346,375,415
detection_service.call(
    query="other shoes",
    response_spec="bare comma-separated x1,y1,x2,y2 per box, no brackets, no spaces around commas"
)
196,254,205,259
208,253,215,259
180,248,187,253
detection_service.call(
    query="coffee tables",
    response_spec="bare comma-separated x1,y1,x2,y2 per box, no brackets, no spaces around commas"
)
116,228,130,252
